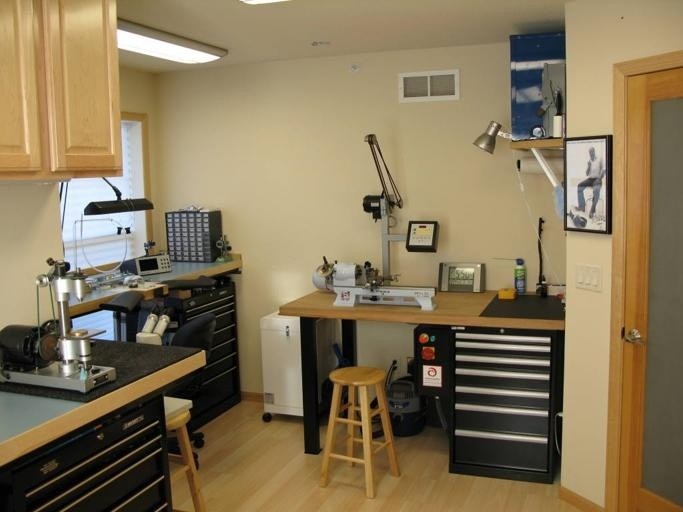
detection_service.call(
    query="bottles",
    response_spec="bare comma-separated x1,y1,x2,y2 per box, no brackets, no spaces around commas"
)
513,259,526,293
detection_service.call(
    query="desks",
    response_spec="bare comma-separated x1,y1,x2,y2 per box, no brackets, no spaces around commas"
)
280,287,565,484
1,339,206,512
67,252,242,434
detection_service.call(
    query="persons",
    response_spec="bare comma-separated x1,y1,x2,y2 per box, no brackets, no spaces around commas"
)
574,147,606,220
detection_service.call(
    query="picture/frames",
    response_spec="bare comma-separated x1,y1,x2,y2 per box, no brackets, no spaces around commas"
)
564,134,612,233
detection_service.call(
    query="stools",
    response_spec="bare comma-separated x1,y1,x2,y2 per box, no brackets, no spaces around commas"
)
163,395,208,512
318,366,401,499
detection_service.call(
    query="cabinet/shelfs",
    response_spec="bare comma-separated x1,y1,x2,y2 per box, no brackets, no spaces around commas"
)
1,0,124,181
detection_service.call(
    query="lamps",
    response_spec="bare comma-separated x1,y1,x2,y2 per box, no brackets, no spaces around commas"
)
117,18,228,64
59,177,154,216
473,121,512,152
